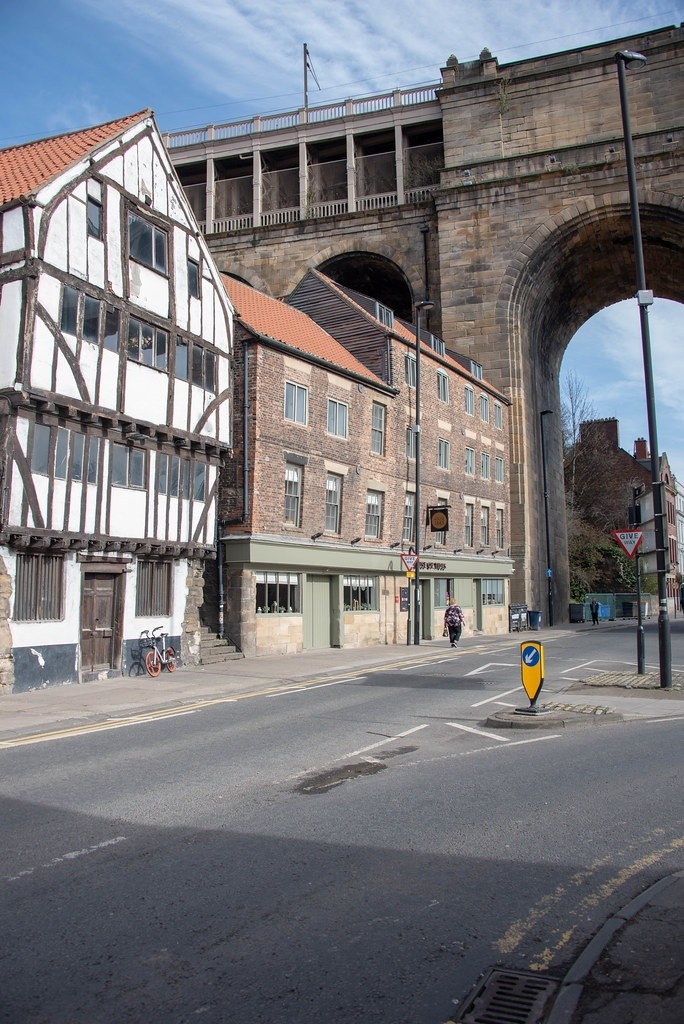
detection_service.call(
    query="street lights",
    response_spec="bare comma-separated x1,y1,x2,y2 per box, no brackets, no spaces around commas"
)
614,49,678,685
412,300,435,644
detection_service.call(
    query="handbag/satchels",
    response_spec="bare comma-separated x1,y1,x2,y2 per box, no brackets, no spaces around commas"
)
443,627,448,637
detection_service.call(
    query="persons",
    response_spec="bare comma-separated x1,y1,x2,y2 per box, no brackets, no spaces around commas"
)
590,599,599,625
444,599,466,647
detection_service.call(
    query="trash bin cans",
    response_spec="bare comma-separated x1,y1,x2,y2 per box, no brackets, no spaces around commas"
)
622,602,638,620
508,604,527,633
569,604,586,623
527,610,542,631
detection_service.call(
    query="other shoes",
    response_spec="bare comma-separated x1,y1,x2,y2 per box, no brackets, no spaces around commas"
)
450,642,457,649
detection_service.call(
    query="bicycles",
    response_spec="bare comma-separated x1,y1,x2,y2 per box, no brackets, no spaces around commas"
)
137,626,176,677
